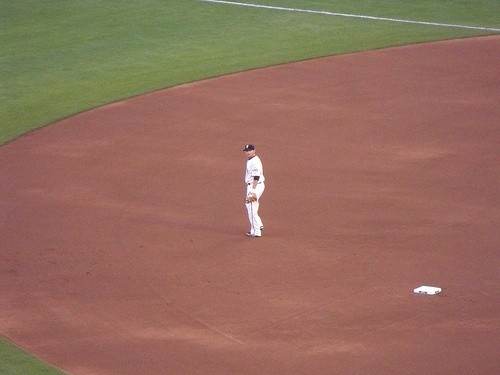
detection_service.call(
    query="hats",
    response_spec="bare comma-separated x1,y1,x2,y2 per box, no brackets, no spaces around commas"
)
244,144,255,151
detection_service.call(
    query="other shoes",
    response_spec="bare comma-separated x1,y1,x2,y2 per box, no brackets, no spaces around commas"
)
260,226,264,230
246,232,258,237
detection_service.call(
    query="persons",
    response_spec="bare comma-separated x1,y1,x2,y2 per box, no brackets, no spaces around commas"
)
241,145,266,236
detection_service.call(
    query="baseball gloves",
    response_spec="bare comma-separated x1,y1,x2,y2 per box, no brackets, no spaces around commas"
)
247,193,257,203
